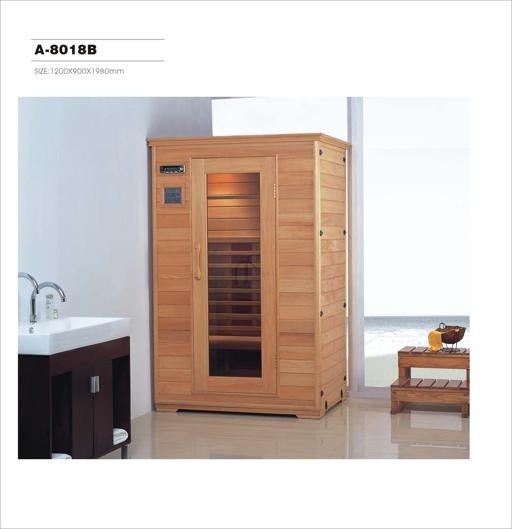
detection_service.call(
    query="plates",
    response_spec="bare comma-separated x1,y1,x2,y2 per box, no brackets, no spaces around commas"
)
18,335,131,459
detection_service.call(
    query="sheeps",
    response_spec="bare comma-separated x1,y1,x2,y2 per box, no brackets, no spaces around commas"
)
18,273,40,294
25,281,66,323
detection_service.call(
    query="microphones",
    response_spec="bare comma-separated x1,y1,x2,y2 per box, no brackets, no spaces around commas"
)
390,378,469,418
